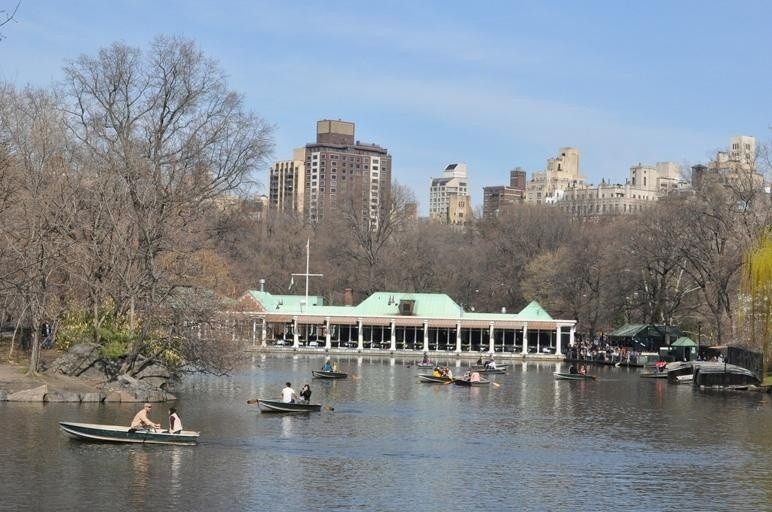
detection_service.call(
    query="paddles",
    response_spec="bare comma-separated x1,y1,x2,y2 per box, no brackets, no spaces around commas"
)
128,427,153,433
247,397,282,404
444,375,469,384
299,398,334,411
479,377,500,386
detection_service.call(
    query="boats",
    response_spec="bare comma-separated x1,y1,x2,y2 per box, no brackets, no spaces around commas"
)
257,399,322,413
312,370,348,379
57,421,201,446
415,360,509,386
551,371,598,381
639,370,668,378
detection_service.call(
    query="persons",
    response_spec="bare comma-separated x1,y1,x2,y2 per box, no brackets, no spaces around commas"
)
130,403,161,434
422,356,433,365
656,359,666,371
297,384,312,405
42,321,52,349
281,382,298,404
331,361,339,373
434,366,453,380
697,351,718,361
321,360,334,372
162,407,183,434
477,356,496,370
565,339,642,376
